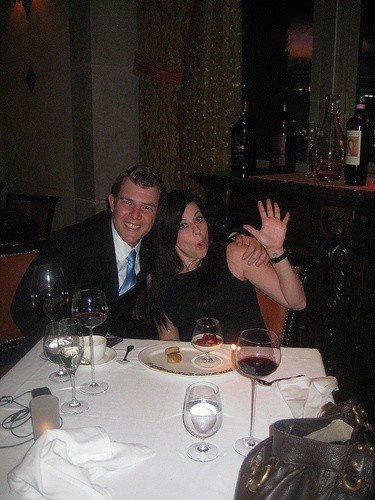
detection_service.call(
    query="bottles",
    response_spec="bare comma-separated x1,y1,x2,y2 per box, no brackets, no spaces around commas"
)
309,89,371,187
230,98,254,176
269,87,296,175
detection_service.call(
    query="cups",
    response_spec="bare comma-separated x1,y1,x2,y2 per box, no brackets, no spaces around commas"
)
29,395,60,440
79,335,107,363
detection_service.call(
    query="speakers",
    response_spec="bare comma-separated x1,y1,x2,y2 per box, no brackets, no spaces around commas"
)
27,385,59,438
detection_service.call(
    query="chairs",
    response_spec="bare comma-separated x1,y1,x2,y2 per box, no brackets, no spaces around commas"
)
5,192,62,237
257,262,313,348
0,240,42,377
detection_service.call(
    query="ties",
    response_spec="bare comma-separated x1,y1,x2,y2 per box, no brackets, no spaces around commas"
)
118,249,137,295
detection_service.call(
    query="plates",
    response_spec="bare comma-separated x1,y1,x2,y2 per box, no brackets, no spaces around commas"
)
79,346,115,365
139,340,238,376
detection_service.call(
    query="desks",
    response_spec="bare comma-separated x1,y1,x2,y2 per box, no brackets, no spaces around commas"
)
0,331,337,500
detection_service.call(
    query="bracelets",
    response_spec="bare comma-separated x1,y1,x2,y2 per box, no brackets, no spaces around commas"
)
269,253,288,265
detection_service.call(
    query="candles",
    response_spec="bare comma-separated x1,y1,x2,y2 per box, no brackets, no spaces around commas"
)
28,394,60,440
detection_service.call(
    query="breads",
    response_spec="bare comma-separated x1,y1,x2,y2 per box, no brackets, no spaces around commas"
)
165,346,182,364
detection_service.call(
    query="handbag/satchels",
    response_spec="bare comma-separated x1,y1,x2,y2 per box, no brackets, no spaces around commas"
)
234,398,374,500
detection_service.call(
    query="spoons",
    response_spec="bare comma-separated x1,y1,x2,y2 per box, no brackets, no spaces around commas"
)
116,345,135,364
256,374,304,386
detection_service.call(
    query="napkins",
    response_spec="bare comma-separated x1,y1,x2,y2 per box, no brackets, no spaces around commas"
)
277,375,339,418
6,427,156,500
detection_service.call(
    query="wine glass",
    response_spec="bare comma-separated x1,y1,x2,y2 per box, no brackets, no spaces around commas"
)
29,263,72,360
71,288,110,393
43,318,89,416
233,327,281,457
183,382,223,461
191,317,226,368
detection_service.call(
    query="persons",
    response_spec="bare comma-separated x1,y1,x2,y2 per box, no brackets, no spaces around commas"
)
10,165,163,347
144,185,307,347
